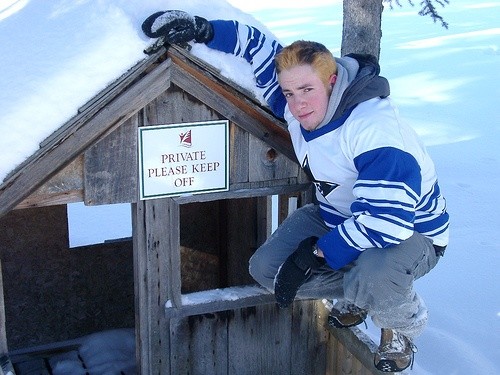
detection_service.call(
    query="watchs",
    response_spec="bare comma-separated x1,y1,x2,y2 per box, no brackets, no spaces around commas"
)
312,243,318,256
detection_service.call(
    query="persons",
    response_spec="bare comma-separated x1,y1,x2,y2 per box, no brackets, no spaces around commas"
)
141,9,450,372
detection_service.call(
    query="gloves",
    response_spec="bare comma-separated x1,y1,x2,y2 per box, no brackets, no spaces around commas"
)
141,10,215,55
274,236,328,308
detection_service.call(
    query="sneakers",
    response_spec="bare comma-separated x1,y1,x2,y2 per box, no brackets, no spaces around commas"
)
327,298,368,330
374,329,418,372
